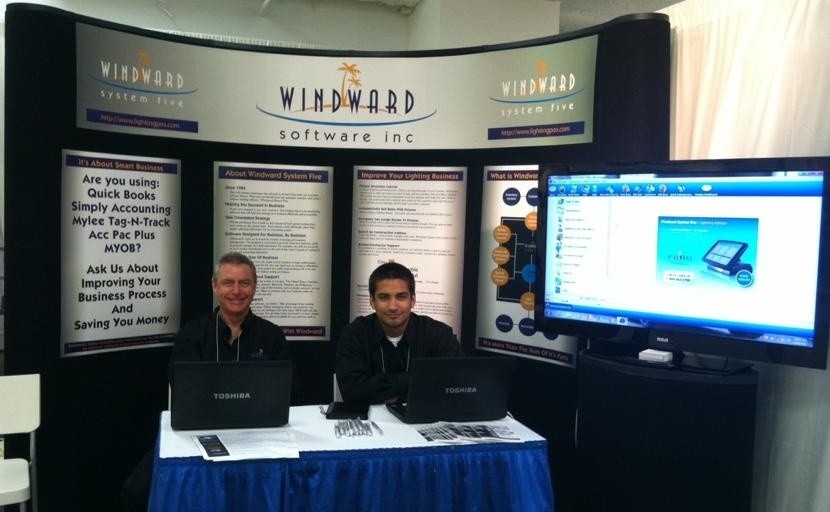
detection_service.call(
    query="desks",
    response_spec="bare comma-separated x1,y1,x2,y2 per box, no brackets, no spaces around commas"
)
150,410,550,512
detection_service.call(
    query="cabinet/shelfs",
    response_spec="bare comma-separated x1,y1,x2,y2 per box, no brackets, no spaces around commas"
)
573,347,758,510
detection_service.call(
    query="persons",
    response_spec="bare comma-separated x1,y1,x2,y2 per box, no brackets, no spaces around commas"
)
332,262,464,408
169,252,288,390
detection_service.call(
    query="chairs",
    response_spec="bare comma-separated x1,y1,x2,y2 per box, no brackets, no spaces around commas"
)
0,372,44,511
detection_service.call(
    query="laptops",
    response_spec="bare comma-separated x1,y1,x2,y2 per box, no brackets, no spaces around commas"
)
386,356,516,424
171,360,293,430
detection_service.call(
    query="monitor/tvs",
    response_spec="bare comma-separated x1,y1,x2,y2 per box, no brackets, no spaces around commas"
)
534,156,830,375
703,240,748,270
